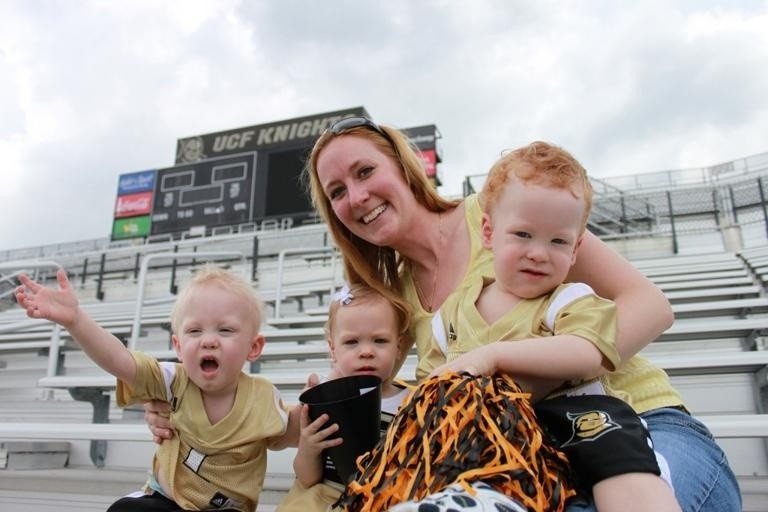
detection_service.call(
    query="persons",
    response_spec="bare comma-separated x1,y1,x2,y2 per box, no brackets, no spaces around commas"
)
15,268,318,511
146,115,745,512
416,141,685,512
275,283,417,512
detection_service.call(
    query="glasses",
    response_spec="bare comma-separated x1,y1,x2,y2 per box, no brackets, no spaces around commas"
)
329,115,392,144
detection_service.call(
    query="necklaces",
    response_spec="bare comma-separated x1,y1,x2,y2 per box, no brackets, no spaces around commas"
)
411,210,442,312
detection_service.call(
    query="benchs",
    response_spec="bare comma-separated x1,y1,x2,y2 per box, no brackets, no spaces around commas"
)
626,247,768,511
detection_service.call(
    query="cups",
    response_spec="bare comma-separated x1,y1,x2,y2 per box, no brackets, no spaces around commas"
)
298,374,384,483
